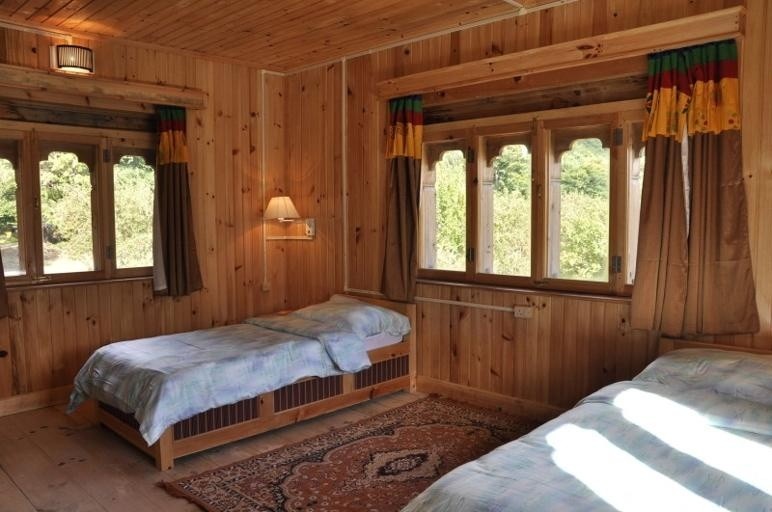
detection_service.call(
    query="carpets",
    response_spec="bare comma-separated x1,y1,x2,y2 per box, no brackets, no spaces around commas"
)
152,394,543,512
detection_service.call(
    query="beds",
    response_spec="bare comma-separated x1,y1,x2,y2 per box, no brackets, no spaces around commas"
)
93,291,416,472
429,335,771,512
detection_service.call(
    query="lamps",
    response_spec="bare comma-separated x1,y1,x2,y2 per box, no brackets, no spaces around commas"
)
261,196,300,222
50,46,94,75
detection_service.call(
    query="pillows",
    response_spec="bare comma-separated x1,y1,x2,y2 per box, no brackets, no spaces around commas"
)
291,301,390,338
633,346,771,403
